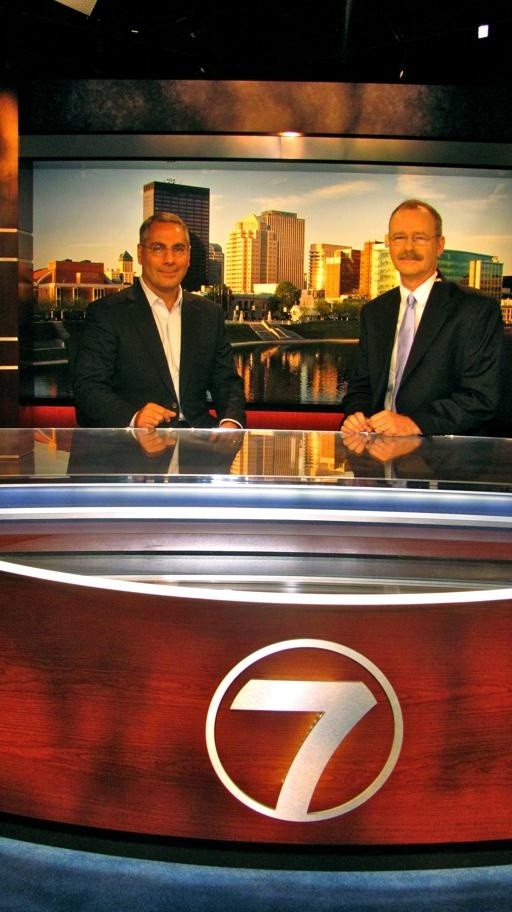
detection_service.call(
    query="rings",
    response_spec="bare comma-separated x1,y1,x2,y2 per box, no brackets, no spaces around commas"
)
380,425,387,433
379,442,385,448
233,440,241,444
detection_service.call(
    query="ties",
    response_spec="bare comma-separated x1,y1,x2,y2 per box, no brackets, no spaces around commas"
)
391,294,416,416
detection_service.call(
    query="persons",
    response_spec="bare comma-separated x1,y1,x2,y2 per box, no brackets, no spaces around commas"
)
341,435,502,490
340,201,499,436
69,428,239,476
73,211,246,455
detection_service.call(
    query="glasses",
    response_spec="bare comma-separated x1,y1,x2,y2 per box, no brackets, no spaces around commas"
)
138,245,192,257
387,234,440,244
